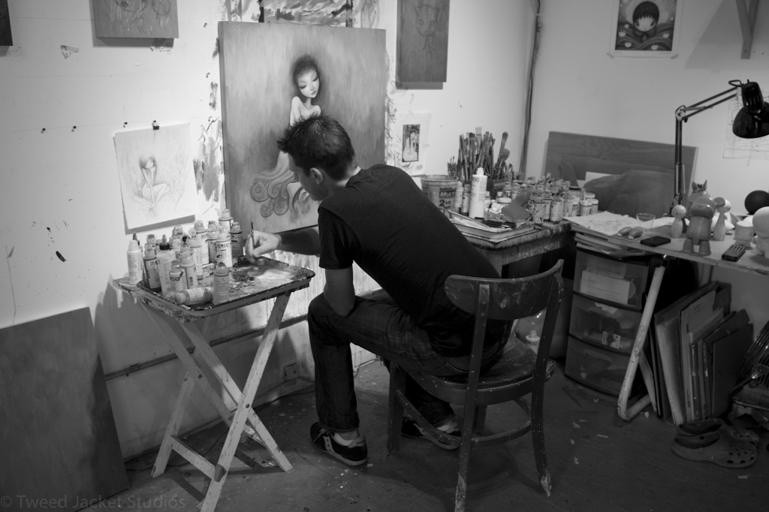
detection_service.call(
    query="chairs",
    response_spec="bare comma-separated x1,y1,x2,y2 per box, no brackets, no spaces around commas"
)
386,260,565,511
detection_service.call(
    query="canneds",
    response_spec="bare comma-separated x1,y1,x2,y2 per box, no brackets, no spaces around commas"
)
530,187,599,224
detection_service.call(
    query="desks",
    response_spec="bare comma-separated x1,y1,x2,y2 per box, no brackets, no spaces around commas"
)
111,257,315,512
609,217,768,421
449,206,604,277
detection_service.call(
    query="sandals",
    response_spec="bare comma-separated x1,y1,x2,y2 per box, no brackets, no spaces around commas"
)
677,416,761,446
670,430,759,469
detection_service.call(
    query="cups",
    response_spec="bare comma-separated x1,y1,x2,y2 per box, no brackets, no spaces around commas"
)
635,212,656,238
735,221,754,248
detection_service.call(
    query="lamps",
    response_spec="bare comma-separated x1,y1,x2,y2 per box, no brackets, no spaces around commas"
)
667,80,768,235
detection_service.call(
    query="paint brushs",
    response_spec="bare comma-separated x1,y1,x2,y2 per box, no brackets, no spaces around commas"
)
457,127,516,183
250,221,259,270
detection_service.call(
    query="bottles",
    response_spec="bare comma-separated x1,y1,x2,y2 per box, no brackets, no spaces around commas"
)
128,208,242,305
454,167,599,221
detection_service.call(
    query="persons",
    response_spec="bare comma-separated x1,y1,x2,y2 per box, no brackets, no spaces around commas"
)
402,130,418,161
289,54,322,127
277,109,511,467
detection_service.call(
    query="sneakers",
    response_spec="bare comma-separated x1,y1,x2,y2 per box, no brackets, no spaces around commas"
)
400,415,463,451
310,421,369,466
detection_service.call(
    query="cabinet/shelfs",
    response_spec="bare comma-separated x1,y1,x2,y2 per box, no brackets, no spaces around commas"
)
564,249,650,399
